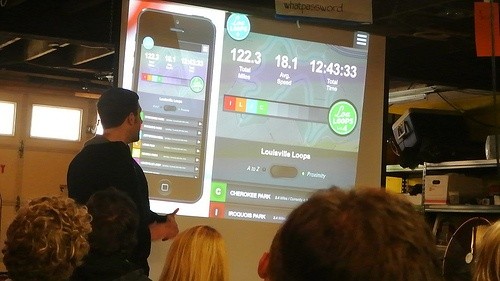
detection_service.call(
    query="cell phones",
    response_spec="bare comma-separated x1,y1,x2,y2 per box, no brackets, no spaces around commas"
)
128,8,216,204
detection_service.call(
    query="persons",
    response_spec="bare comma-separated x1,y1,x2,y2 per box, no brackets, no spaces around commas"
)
159,225,229,281
257,183,439,281
1,195,94,281
65,88,180,281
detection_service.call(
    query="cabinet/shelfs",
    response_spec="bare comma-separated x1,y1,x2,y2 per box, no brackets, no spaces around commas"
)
386,159,500,251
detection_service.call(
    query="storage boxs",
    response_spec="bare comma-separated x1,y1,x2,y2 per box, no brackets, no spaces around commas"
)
424,174,465,205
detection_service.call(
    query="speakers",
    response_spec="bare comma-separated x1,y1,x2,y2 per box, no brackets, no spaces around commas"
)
392,108,472,151
